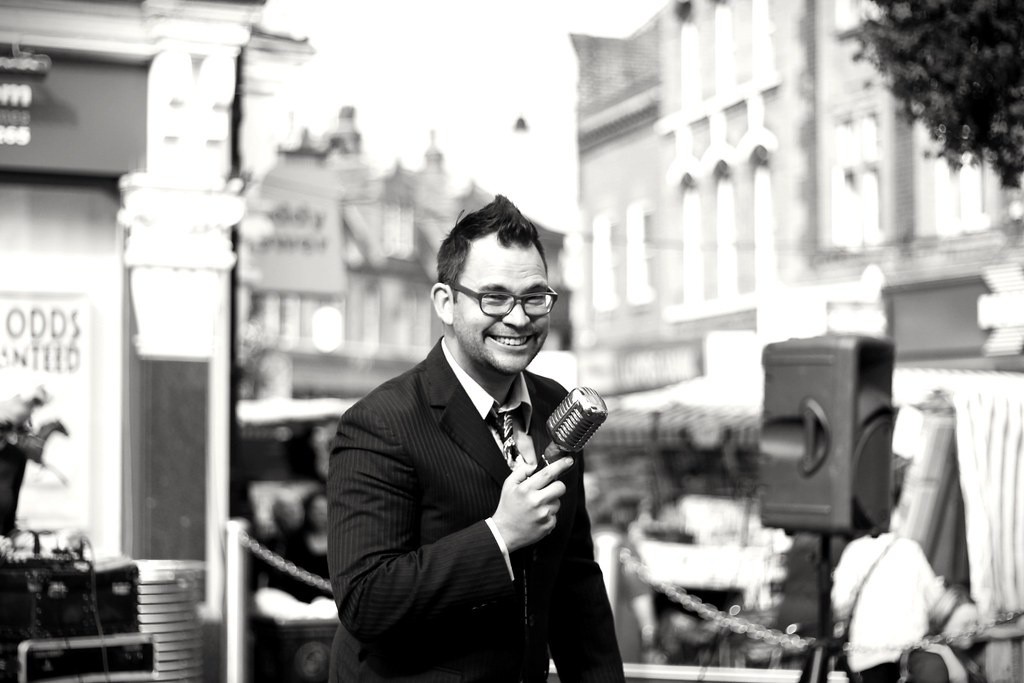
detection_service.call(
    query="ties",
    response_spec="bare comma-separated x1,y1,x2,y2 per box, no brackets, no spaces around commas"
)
488,407,526,469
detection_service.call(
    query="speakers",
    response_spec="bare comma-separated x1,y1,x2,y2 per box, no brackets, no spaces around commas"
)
0,635,159,683
757,334,897,537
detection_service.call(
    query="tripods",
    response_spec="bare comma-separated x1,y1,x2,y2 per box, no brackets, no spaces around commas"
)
798,533,854,683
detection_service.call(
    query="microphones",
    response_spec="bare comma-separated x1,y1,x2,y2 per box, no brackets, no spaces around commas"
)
528,388,608,478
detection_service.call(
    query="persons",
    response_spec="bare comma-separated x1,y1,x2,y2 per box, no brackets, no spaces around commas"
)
831,531,945,683
328,195,625,683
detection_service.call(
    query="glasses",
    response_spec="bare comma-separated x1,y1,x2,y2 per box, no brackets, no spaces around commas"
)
451,282,559,319
